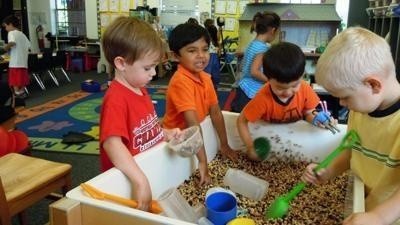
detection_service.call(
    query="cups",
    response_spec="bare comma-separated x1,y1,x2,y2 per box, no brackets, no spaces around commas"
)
204,192,238,225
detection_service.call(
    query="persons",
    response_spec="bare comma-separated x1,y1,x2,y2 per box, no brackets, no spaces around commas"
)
99,16,181,211
204,19,222,55
0,106,29,158
300,26,400,225
230,11,282,113
207,25,221,86
236,42,338,160
3,13,32,99
162,22,239,189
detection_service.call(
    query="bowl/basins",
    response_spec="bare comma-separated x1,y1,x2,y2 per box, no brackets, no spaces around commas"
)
250,136,272,162
167,125,205,159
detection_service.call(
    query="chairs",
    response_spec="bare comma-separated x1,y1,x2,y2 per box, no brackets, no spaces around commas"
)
1,151,72,225
38,48,60,86
53,50,71,83
28,54,46,90
